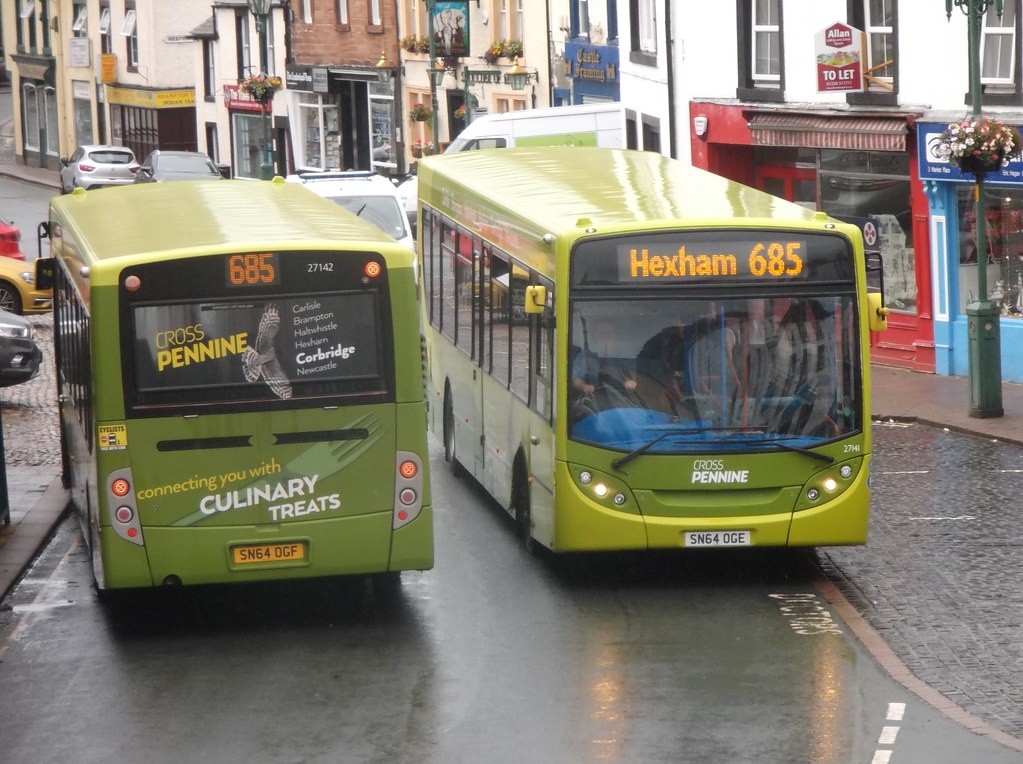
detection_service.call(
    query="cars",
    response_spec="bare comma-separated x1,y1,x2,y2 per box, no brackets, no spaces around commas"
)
0,216,54,389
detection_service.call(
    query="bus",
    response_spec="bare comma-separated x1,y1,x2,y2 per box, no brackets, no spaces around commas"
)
416,146,889,557
34,176,435,600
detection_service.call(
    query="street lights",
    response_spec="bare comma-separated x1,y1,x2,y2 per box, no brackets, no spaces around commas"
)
240,0,282,180
422,0,446,154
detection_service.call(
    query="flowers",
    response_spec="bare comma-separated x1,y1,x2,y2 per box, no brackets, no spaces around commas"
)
484,40,523,64
938,116,1023,167
241,75,282,105
454,106,466,121
411,138,435,150
396,34,430,53
410,104,433,128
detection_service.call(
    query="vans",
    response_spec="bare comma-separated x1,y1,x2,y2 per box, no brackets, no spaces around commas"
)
396,101,621,241
285,167,418,287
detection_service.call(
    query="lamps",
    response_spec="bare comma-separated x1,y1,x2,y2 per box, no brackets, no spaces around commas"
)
425,59,447,86
505,55,538,90
375,52,406,83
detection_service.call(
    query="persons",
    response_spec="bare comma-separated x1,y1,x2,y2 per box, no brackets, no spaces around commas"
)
662,302,743,424
570,319,639,393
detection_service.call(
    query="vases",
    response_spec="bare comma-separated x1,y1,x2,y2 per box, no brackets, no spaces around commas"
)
411,146,434,158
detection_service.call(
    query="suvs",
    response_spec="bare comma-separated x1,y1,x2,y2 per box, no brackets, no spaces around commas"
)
133,150,226,184
59,145,141,196
